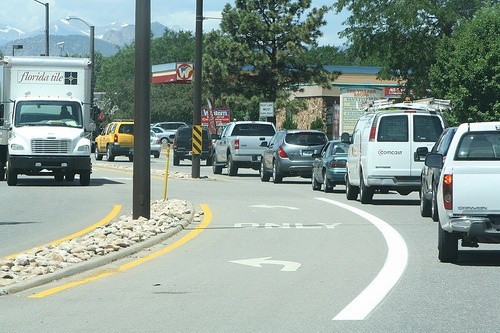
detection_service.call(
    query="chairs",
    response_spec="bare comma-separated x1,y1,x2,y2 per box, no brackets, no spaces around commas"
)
466,135,496,158
335,147,345,153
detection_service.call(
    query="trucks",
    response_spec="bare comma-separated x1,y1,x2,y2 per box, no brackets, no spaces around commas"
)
341,108,445,205
0,55,96,185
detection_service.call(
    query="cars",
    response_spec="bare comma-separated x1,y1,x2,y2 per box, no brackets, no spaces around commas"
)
150,126,176,144
150,130,162,159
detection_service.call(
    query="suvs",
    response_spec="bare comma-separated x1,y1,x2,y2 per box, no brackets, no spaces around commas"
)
211,121,277,177
173,124,214,166
311,139,353,192
154,122,188,132
424,121,499,260
414,126,461,220
259,129,330,184
95,118,135,161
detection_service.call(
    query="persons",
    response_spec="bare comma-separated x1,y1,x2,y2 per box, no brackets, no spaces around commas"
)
60,105,71,118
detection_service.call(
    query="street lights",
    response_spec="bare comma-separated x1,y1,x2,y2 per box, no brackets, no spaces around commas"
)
66,17,95,107
34,0,50,56
